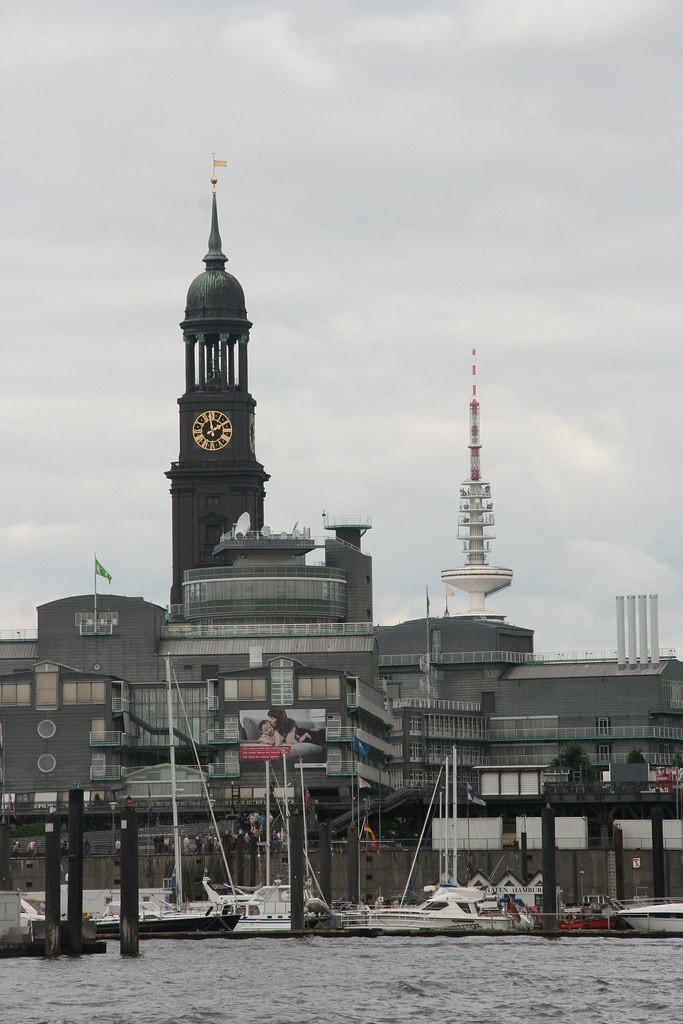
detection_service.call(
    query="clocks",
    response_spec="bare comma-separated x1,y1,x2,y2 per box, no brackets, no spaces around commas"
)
249,412,256,454
191,410,232,450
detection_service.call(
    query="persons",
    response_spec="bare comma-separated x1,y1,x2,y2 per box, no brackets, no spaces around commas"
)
153,835,164,853
115,837,121,856
83,838,90,857
206,812,280,852
182,834,192,853
257,710,325,746
12,838,21,860
27,839,39,859
193,833,202,853
59,836,65,856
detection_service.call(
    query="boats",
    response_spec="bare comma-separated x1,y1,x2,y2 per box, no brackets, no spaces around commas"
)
24,650,682,945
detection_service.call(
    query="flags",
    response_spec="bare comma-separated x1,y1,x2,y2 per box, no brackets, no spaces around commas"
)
466,780,487,806
94,558,112,586
358,776,372,789
363,818,377,849
304,784,318,821
